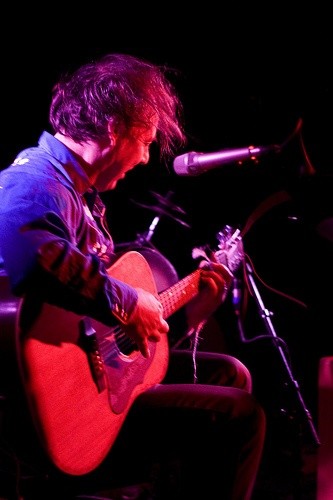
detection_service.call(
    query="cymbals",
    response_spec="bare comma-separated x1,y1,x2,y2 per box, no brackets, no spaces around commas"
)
152,191,186,214
133,201,191,230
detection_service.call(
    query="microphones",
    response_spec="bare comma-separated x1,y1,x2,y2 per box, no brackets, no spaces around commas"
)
172,145,283,176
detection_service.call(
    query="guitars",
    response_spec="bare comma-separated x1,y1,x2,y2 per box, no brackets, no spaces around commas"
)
0,224,245,478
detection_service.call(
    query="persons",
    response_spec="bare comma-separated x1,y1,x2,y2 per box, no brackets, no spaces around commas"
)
0,53,266,500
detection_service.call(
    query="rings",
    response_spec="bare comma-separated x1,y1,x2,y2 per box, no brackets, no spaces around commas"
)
221,286,228,292
